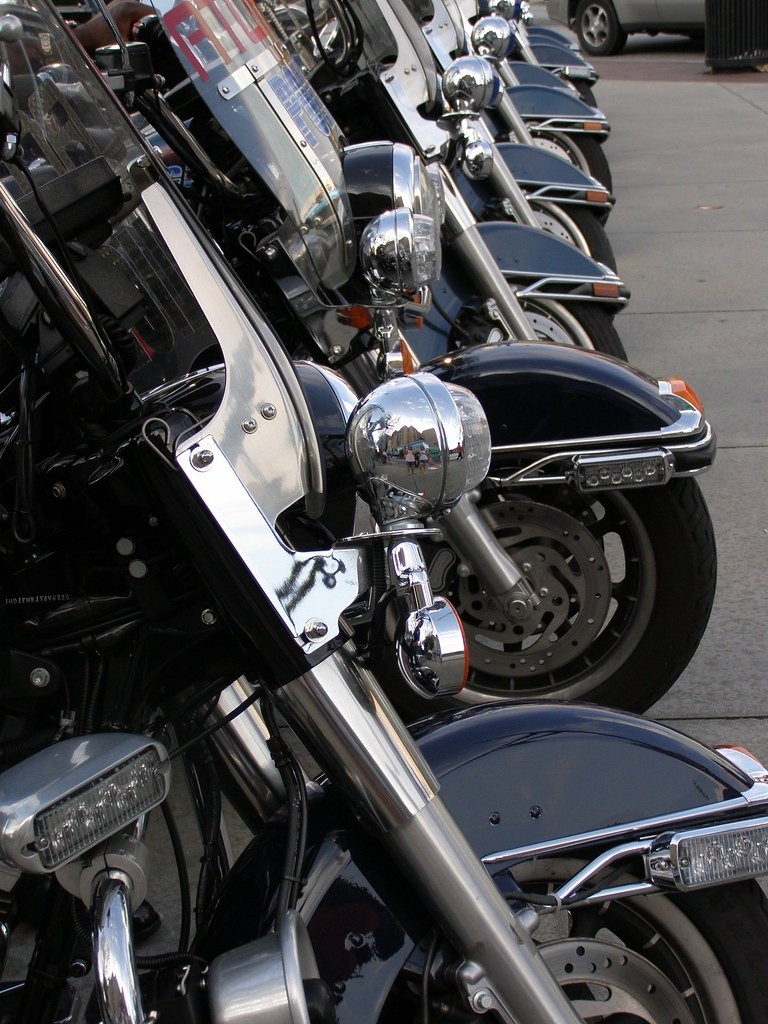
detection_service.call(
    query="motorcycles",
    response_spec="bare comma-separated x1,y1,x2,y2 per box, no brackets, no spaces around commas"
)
0,0,720,716
0,0,767,1023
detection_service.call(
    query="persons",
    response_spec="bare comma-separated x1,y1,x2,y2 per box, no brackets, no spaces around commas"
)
373,447,387,465
453,443,463,460
418,445,428,474
404,445,416,475
0,0,161,76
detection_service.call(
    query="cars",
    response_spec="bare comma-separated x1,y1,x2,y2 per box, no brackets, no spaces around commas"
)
545,0,708,59
